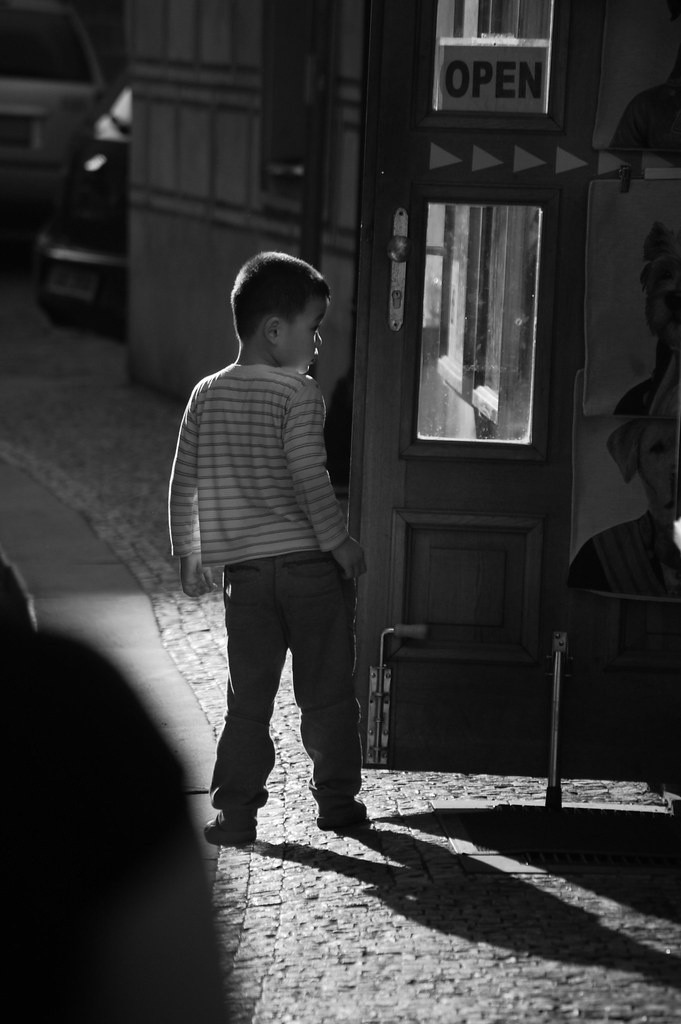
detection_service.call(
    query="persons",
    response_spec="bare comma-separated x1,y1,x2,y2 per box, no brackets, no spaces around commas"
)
167,249,369,843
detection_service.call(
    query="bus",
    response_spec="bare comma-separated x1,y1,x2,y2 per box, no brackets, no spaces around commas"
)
128,0,680,810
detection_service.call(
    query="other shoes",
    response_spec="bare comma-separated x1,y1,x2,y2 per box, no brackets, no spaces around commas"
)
204,815,258,843
318,798,368,828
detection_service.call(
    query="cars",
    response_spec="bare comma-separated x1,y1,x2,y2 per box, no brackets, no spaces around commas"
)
33,90,131,336
0,79,95,208
